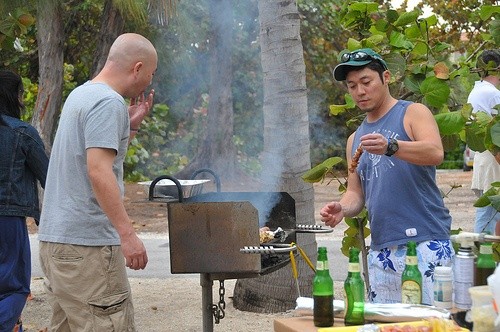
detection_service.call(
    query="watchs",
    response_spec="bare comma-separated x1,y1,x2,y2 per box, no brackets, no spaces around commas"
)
384,138,399,156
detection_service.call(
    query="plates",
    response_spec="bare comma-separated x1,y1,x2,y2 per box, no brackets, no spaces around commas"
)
317,318,471,332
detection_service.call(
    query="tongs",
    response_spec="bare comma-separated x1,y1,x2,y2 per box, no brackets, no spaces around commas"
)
290,242,318,298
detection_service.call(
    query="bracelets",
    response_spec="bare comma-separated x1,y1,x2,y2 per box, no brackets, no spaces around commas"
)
131,128,137,131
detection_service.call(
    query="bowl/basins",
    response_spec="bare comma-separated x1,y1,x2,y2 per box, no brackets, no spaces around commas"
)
137,178,210,199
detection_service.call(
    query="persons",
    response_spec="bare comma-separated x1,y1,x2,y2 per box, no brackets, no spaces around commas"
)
320,48,457,308
467,49,500,236
0,70,49,332
39,33,158,332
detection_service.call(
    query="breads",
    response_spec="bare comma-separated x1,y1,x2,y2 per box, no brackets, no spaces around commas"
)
259,227,275,243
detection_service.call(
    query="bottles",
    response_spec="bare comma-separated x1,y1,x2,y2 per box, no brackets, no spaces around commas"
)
344,249,364,326
451,230,500,265
432,267,453,309
313,246,334,327
475,243,497,287
400,242,422,305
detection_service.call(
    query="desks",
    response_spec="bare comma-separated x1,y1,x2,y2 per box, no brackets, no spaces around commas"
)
274,315,389,332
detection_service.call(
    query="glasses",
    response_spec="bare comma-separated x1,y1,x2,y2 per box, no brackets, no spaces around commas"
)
340,52,376,62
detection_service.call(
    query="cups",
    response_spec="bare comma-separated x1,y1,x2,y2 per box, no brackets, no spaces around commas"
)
468,285,500,332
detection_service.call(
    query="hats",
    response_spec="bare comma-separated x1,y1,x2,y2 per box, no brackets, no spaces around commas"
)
333,48,389,81
476,50,500,78
